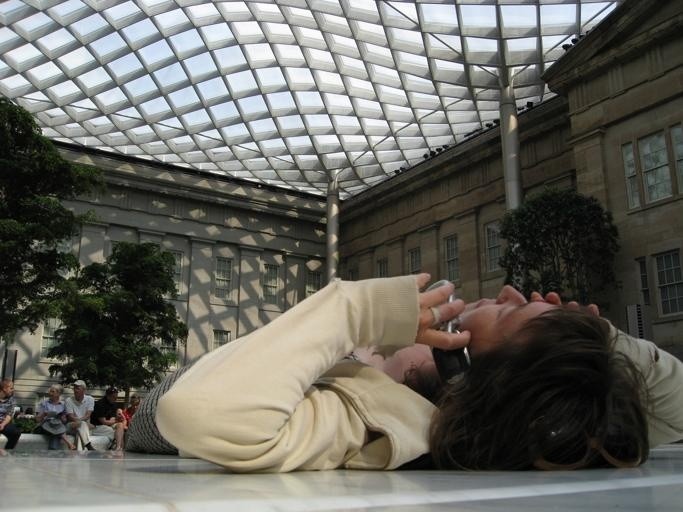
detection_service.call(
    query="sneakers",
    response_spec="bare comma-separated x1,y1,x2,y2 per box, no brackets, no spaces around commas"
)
69,441,96,451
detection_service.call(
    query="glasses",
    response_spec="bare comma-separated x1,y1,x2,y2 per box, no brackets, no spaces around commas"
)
522,410,644,473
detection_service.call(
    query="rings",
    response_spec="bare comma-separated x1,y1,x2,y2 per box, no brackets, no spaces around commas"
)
432,305,441,326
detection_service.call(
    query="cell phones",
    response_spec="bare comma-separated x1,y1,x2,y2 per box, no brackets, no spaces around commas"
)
419,279,474,397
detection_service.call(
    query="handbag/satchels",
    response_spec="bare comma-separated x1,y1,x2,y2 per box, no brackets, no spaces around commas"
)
40,417,66,435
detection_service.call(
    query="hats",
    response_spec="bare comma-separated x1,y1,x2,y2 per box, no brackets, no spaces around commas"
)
70,379,87,390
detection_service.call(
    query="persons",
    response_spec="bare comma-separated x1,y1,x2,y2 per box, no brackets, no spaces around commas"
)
34,383,77,450
110,408,128,430
65,379,97,451
0,377,23,457
118,270,683,476
123,393,142,426
91,386,125,453
